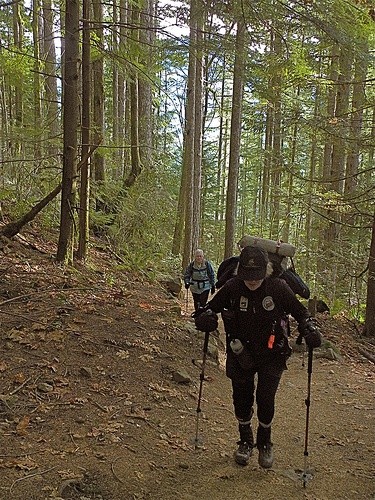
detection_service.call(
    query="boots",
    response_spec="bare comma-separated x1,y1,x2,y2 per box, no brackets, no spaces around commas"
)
257,427,273,469
234,428,254,466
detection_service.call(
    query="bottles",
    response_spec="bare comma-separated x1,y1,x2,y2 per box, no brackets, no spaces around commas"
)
227,334,243,355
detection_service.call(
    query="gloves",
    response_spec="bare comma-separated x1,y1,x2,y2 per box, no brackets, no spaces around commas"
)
185,282,190,289
211,287,215,295
193,312,219,332
298,323,321,348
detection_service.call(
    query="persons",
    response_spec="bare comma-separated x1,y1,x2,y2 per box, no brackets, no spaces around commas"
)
183,249,215,312
193,245,322,467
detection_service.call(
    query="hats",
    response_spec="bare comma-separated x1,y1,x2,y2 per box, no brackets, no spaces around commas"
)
237,246,268,280
